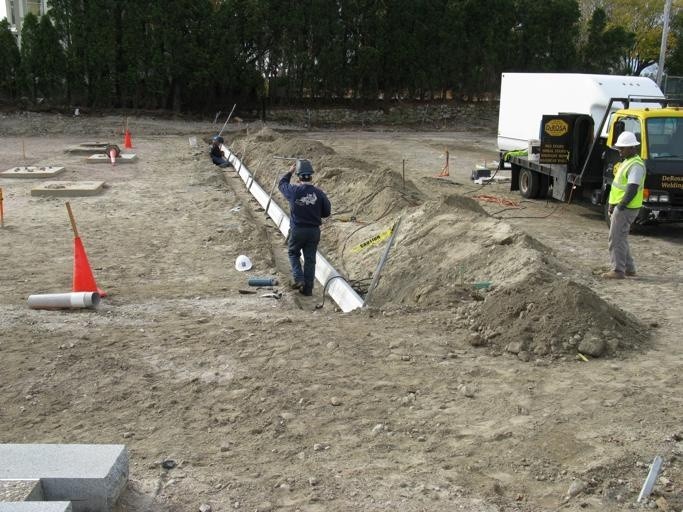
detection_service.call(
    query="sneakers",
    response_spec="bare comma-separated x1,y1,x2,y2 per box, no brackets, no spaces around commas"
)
626,270,636,275
300,287,312,296
601,270,626,280
291,281,305,290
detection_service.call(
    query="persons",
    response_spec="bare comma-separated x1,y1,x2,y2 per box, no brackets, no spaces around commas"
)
210,136,229,167
595,131,646,280
278,158,331,295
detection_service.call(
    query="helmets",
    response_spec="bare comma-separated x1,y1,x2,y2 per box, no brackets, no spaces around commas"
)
213,136,223,143
615,131,641,149
294,158,315,175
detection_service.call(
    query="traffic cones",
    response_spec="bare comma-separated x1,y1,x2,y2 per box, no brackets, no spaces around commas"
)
103,143,120,166
70,235,106,298
123,126,133,148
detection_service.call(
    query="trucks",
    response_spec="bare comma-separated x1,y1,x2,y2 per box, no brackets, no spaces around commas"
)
492,69,669,160
502,94,682,234
